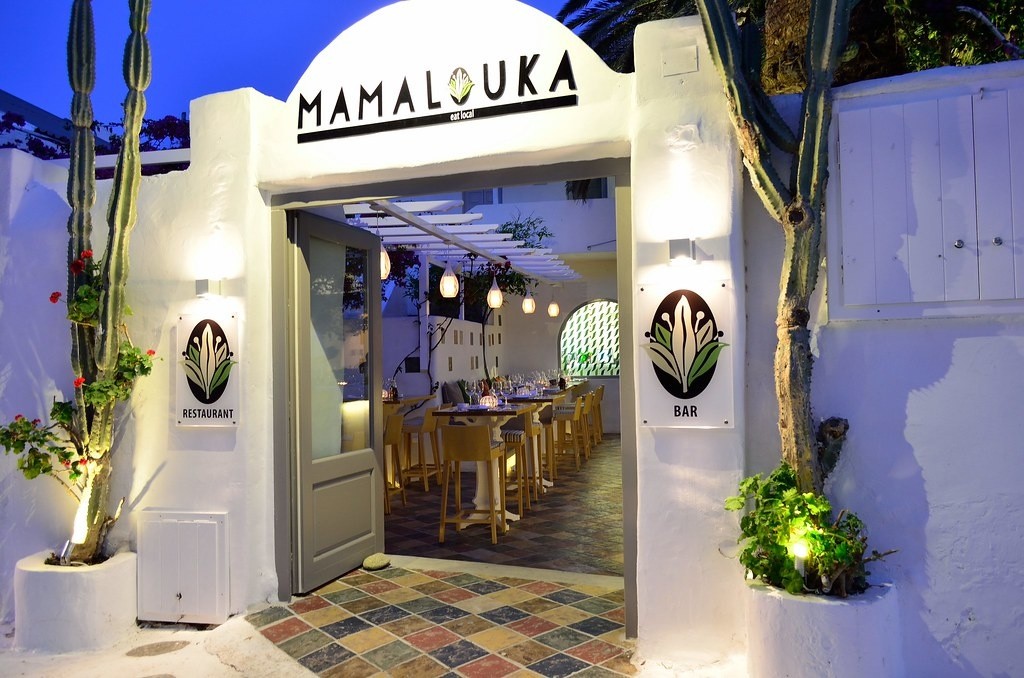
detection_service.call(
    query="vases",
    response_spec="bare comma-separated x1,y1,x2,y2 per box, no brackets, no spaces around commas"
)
14,549,136,655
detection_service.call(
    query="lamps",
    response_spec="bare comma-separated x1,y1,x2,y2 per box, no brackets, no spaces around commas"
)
548,288,560,317
376,211,391,279
440,245,460,298
521,283,536,314
486,265,503,308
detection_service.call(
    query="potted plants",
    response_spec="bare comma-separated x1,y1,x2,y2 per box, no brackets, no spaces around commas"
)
722,467,905,678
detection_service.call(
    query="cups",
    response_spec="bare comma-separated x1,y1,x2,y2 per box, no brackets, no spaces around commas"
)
457,403,468,412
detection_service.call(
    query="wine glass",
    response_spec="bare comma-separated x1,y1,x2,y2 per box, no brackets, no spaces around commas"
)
465,369,560,410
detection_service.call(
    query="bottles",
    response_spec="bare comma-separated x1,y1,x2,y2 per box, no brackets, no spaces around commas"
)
390,380,398,397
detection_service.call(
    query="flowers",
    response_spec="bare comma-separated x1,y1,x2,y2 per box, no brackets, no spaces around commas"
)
0,250,161,565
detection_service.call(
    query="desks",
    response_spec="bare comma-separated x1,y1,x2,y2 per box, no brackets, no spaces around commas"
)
429,399,537,530
500,392,565,490
566,382,583,434
572,378,589,395
345,394,437,403
541,384,574,465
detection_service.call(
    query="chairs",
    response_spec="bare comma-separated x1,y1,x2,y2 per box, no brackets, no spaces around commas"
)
382,384,604,545
